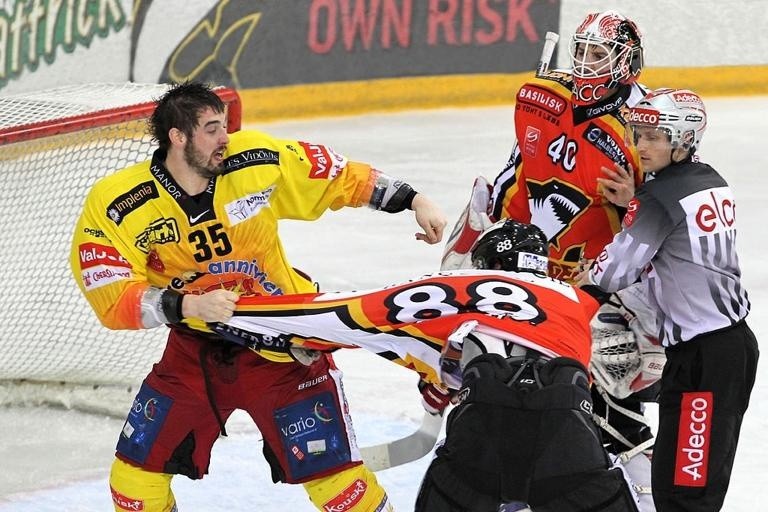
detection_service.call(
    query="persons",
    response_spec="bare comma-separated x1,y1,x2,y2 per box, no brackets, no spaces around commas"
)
225,218,642,512
576,89,761,511
420,10,653,511
71,82,447,511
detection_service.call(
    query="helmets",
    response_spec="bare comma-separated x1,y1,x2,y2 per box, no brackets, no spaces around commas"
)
568,10,644,106
470,218,550,276
622,87,708,152
